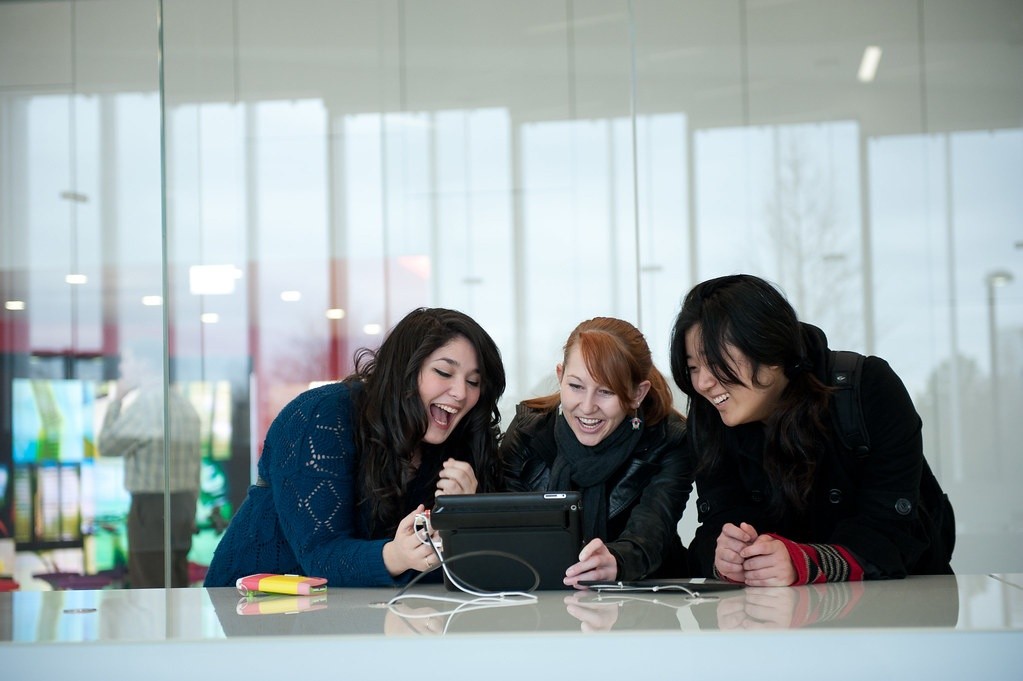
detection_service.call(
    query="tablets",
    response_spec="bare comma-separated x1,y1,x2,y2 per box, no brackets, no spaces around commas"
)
576,578,745,591
431,492,582,593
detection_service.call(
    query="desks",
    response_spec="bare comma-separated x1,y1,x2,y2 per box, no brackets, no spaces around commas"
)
0,572,1023,680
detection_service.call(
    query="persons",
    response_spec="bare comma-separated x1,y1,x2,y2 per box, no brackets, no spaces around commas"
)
500,317,695,590
207,581,958,637
204,308,506,588
99,346,201,588
671,274,955,586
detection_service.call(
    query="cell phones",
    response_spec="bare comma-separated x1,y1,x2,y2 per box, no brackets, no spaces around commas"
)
236,573,328,596
236,593,328,615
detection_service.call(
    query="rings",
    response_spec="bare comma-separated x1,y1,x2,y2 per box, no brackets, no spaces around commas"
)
426,558,432,569
427,618,429,628
